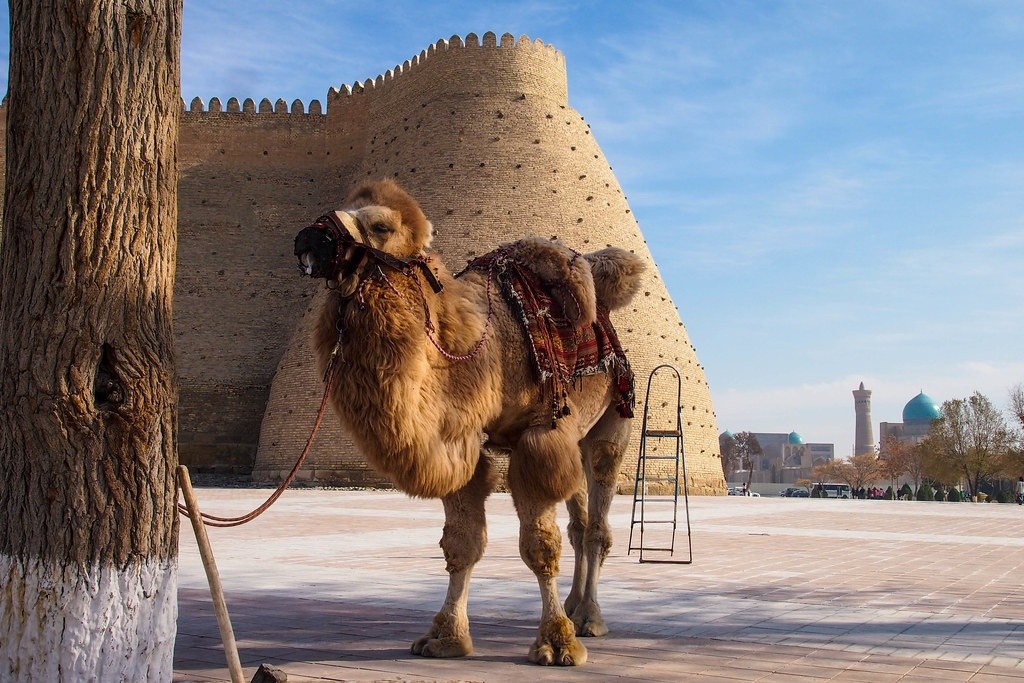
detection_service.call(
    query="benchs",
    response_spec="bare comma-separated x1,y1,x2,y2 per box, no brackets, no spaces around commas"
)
899,494,909,501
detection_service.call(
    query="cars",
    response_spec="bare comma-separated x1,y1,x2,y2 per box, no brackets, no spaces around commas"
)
728,487,760,496
792,490,810,498
779,490,786,497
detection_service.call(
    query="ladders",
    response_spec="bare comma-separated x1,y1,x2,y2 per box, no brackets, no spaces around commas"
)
627,365,695,566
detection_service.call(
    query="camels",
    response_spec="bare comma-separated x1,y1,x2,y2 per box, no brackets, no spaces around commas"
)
294,175,648,666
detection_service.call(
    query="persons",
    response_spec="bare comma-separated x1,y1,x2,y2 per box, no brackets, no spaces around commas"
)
739,474,1024,504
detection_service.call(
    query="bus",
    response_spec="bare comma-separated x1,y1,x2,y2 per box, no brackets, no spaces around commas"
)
810,483,849,499
